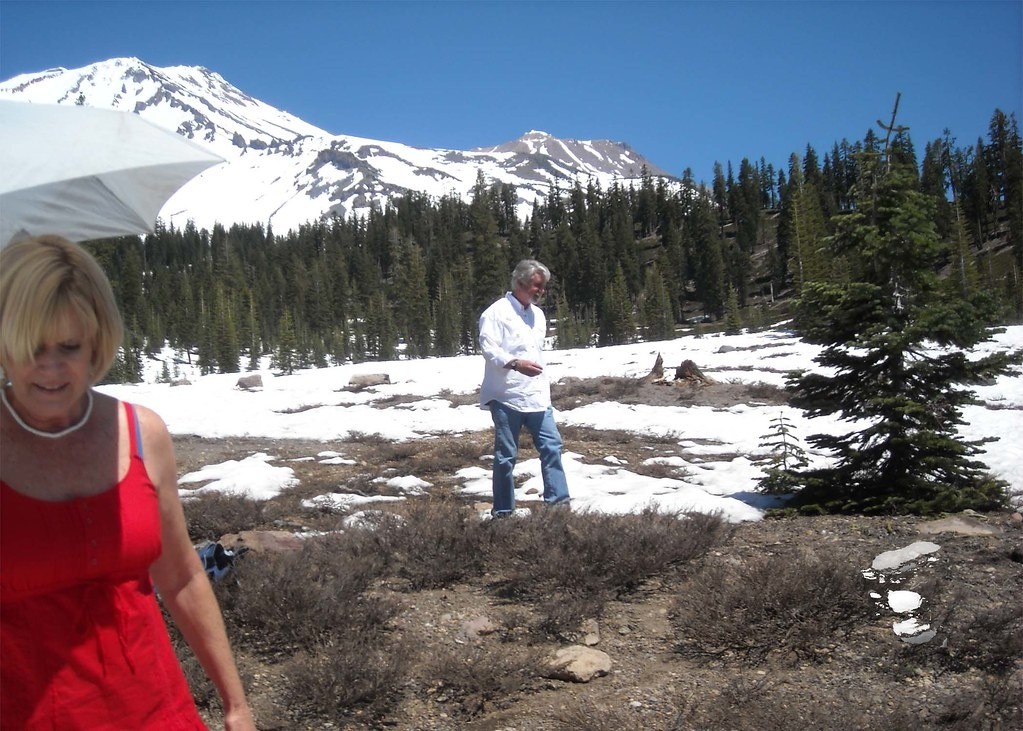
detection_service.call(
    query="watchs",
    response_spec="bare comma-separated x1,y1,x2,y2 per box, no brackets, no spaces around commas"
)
511,359,517,371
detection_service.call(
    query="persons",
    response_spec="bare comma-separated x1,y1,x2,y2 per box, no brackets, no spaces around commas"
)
479,260,570,520
0,235,259,731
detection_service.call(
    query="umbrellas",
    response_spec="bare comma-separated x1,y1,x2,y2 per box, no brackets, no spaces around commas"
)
0,98,230,243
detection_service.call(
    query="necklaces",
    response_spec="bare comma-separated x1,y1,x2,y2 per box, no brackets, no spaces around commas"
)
1,386,93,437
